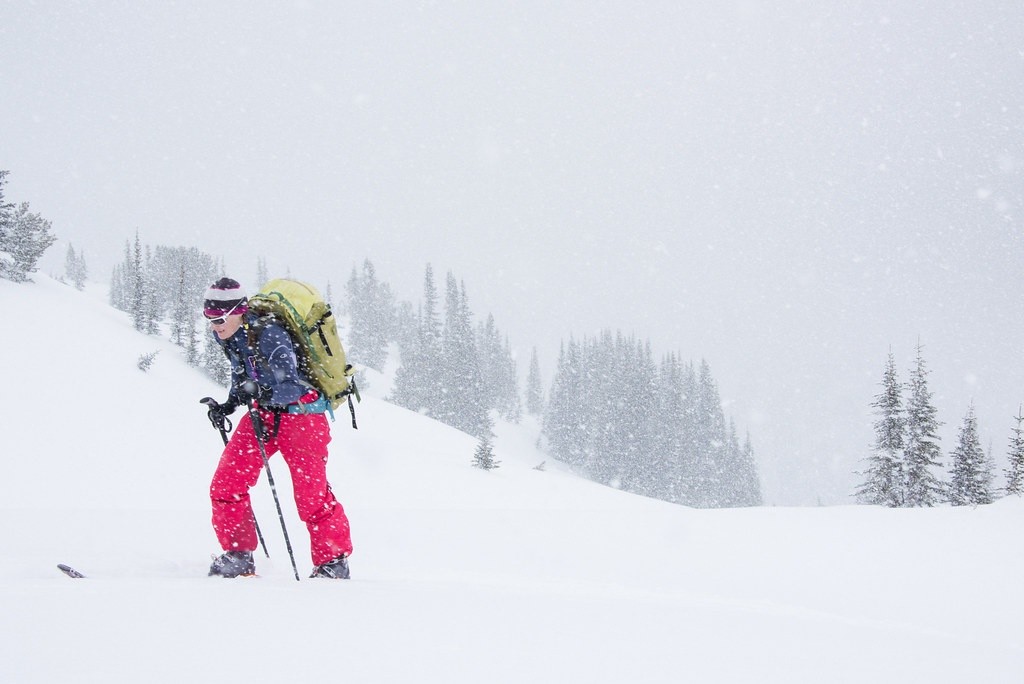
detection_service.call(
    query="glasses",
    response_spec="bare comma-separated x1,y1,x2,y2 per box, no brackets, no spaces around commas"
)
203,298,244,325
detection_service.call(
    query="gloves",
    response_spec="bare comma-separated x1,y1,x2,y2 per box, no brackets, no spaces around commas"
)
238,379,273,401
208,403,234,428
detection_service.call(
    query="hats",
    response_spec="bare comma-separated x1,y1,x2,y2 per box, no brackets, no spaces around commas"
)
204,277,247,315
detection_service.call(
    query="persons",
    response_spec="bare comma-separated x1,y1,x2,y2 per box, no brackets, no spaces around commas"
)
203,276,354,579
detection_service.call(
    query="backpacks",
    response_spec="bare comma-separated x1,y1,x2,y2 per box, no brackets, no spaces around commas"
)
220,278,361,429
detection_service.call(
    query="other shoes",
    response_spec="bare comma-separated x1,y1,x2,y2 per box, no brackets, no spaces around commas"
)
210,551,255,574
309,557,349,579
